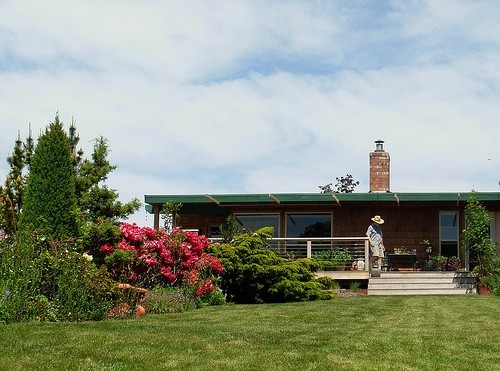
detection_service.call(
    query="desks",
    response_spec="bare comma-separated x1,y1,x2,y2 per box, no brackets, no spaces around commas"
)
388,254,417,271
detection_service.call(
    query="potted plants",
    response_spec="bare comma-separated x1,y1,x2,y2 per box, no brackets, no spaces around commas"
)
324,247,353,271
460,190,500,295
421,240,432,252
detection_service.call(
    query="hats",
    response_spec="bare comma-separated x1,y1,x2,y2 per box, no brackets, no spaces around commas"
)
371,215,384,224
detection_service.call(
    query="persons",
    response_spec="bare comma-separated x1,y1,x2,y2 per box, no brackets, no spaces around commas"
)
366,216,385,270
